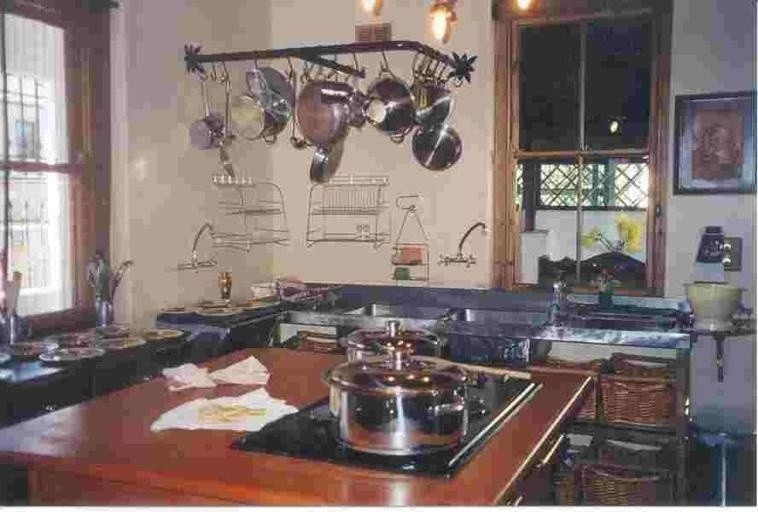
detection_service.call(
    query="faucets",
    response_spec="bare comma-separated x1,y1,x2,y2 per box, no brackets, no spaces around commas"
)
438,222,485,267
179,222,217,271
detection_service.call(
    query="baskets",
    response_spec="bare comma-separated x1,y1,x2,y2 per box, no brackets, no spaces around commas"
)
552,440,661,506
525,352,678,429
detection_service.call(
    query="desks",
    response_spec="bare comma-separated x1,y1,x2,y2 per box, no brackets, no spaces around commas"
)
1,321,190,400
156,298,285,354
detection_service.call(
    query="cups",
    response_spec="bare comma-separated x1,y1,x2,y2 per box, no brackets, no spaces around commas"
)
410,269,417,279
394,268,411,280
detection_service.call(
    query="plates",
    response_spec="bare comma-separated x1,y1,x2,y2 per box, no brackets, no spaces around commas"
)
4,325,185,362
233,300,264,308
199,299,231,307
196,306,241,316
160,306,197,314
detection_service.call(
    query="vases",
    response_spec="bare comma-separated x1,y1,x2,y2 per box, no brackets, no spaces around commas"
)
682,278,748,330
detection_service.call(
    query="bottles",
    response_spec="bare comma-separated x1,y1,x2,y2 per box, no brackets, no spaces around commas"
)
599,268,613,305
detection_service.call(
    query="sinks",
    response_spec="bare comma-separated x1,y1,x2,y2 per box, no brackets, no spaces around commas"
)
443,309,547,325
344,302,451,320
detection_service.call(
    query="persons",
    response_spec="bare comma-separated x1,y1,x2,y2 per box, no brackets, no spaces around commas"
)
696,115,742,179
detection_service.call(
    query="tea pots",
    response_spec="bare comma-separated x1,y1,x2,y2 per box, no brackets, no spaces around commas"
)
93,295,113,328
4,314,31,344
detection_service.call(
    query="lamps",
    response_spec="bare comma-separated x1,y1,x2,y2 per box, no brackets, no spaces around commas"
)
429,0,456,46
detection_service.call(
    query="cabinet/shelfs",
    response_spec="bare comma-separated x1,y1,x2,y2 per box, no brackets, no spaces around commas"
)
210,171,293,251
387,240,430,285
516,228,552,286
303,172,393,251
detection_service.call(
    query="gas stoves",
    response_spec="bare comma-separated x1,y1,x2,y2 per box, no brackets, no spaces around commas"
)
226,365,543,480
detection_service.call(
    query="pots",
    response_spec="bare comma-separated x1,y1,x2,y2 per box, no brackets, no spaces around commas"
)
338,317,443,368
319,354,468,455
187,68,463,185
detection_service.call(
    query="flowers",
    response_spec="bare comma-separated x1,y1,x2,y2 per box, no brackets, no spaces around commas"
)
580,211,644,257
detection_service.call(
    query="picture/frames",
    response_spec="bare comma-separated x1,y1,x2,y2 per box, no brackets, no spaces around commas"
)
672,90,756,197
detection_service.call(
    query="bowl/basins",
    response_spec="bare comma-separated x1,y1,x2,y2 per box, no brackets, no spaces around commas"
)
683,283,746,333
